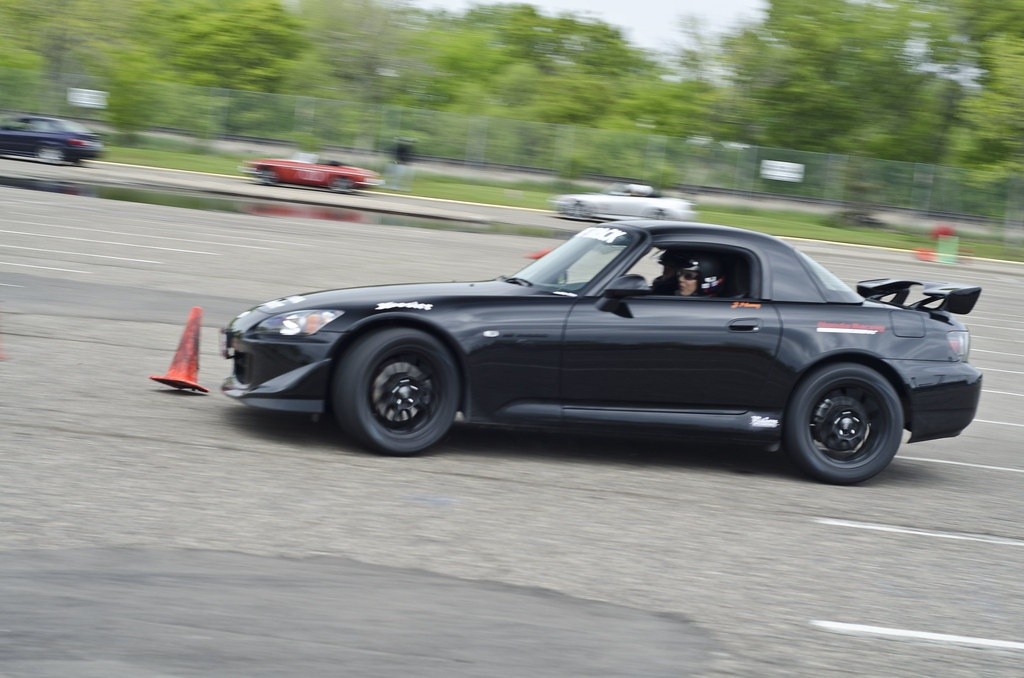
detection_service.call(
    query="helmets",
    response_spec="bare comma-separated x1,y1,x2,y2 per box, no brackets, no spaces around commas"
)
657,250,679,266
677,254,723,296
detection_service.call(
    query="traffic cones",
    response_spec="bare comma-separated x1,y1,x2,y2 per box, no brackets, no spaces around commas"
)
147,306,210,393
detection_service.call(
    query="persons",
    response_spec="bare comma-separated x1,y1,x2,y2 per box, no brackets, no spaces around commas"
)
652,248,723,297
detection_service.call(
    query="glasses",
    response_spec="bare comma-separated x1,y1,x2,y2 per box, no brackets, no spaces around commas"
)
677,268,699,280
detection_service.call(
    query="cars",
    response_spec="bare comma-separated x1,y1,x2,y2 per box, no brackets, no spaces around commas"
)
222,219,985,485
0,115,106,166
546,185,700,229
237,152,384,194
